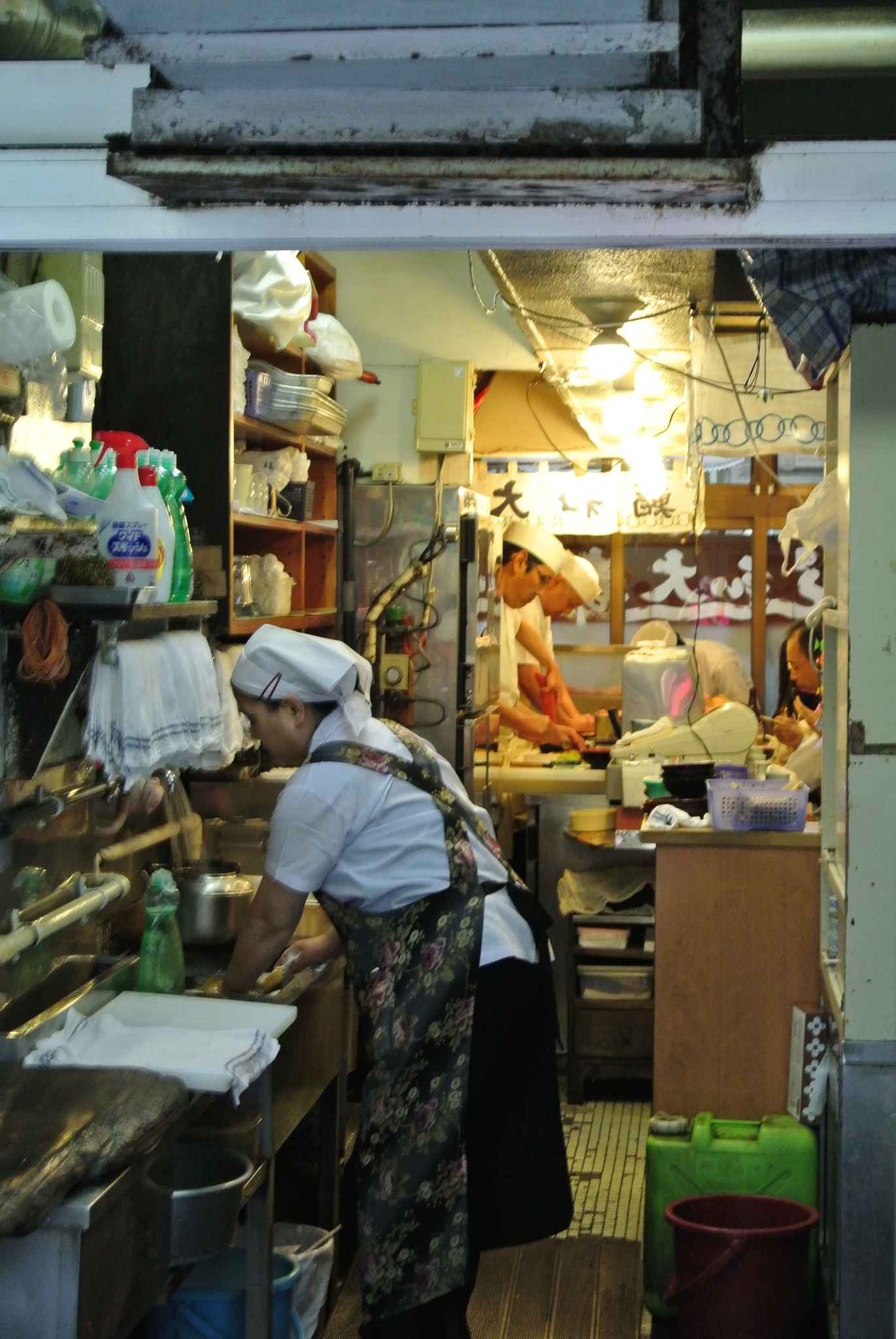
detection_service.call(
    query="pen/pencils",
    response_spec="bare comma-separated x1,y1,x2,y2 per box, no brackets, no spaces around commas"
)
759,715,787,726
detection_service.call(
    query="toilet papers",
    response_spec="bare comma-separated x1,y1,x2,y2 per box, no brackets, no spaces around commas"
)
2,278,79,363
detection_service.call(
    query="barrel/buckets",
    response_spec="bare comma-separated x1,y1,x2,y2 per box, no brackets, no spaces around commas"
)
641,1109,818,1320
663,1193,820,1339
145,1247,304,1339
144,1144,255,1264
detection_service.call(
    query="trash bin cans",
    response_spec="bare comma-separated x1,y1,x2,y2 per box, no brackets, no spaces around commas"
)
232,1223,335,1339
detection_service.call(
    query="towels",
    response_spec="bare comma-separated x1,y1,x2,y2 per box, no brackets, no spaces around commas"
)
85,631,225,794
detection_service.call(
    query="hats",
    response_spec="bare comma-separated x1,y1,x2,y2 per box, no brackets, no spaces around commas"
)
503,521,567,575
631,619,677,647
231,623,371,737
561,555,602,603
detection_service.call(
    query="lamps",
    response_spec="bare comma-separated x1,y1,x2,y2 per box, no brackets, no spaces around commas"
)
572,295,646,384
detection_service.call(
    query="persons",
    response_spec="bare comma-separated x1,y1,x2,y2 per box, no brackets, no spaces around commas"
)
481,522,824,811
221,623,578,1339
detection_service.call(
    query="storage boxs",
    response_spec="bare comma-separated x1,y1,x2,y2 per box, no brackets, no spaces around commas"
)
576,964,655,1003
576,925,631,952
704,774,813,833
786,1001,830,1127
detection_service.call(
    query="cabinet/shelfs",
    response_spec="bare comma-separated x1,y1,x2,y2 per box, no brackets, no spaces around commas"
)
816,316,896,1049
226,246,341,638
561,897,656,1108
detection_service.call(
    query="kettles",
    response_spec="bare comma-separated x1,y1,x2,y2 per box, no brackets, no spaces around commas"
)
134,857,255,946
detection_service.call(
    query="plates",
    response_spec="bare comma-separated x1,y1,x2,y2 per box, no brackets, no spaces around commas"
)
640,794,708,820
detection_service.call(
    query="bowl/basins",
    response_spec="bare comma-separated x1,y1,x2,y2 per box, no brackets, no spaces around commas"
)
580,748,611,770
569,807,617,832
644,761,716,798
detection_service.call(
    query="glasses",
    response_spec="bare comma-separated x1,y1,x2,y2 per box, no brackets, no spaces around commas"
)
533,565,551,587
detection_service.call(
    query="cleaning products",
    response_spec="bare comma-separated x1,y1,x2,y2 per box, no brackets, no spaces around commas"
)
14,863,49,989
138,867,187,994
55,427,197,605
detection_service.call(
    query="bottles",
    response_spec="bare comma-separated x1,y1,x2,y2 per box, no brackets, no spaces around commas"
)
233,554,252,605
138,868,184,994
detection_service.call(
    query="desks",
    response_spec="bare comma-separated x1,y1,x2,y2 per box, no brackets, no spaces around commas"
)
484,742,609,931
636,810,823,1122
